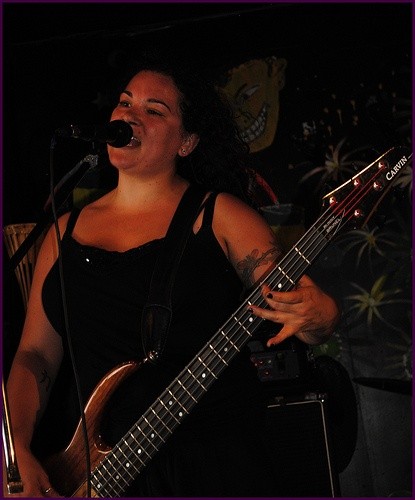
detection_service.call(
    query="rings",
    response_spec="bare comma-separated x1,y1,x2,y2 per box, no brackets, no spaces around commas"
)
41,487,53,494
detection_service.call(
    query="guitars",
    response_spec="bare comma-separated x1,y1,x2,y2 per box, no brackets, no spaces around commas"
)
2,146,415,500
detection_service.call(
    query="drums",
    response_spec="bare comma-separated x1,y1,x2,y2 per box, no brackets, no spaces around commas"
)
3,223,47,315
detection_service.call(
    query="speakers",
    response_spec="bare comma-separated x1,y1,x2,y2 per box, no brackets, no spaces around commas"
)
263,392,335,498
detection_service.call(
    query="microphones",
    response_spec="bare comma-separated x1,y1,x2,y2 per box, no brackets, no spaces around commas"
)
55,120,133,148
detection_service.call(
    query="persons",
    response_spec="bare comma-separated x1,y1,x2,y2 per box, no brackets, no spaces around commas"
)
3,68,340,497
